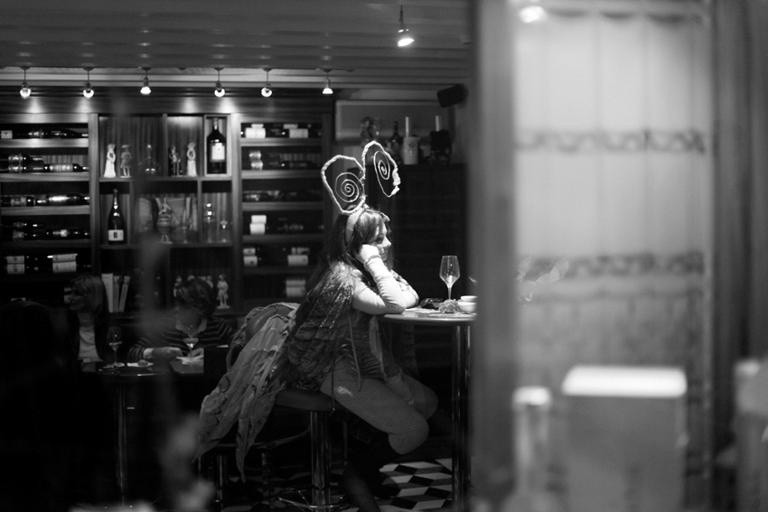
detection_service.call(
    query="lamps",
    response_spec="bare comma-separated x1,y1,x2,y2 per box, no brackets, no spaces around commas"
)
140,67,152,95
322,69,334,94
83,66,94,98
395,0,415,48
214,66,225,97
20,66,31,99
261,67,273,97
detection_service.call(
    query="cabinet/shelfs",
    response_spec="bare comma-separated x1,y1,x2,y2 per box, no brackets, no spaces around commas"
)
0,86,335,343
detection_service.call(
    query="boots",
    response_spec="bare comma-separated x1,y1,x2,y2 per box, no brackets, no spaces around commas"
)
340,431,404,512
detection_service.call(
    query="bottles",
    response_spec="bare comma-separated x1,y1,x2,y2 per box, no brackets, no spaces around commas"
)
9,295,34,303
104,186,127,242
241,122,323,298
387,121,403,156
203,203,229,244
137,142,162,176
206,117,224,173
0,129,89,274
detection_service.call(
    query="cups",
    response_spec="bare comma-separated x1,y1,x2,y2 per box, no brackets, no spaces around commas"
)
401,136,421,166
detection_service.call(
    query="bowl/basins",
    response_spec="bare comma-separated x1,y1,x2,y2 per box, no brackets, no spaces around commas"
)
458,295,478,314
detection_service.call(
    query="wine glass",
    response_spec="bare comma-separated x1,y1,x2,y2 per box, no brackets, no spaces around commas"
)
439,254,460,312
183,337,200,360
103,324,125,370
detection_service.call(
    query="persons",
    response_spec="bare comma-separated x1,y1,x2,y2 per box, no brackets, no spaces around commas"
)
283,205,441,512
128,276,239,509
58,272,130,366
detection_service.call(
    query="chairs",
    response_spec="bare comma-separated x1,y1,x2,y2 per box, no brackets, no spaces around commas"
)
247,305,351,512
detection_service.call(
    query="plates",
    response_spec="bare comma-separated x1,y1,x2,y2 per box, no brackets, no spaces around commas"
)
127,361,155,369
406,309,436,316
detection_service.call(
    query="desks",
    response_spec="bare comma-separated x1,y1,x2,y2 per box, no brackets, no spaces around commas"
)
75,368,160,508
384,307,476,512
